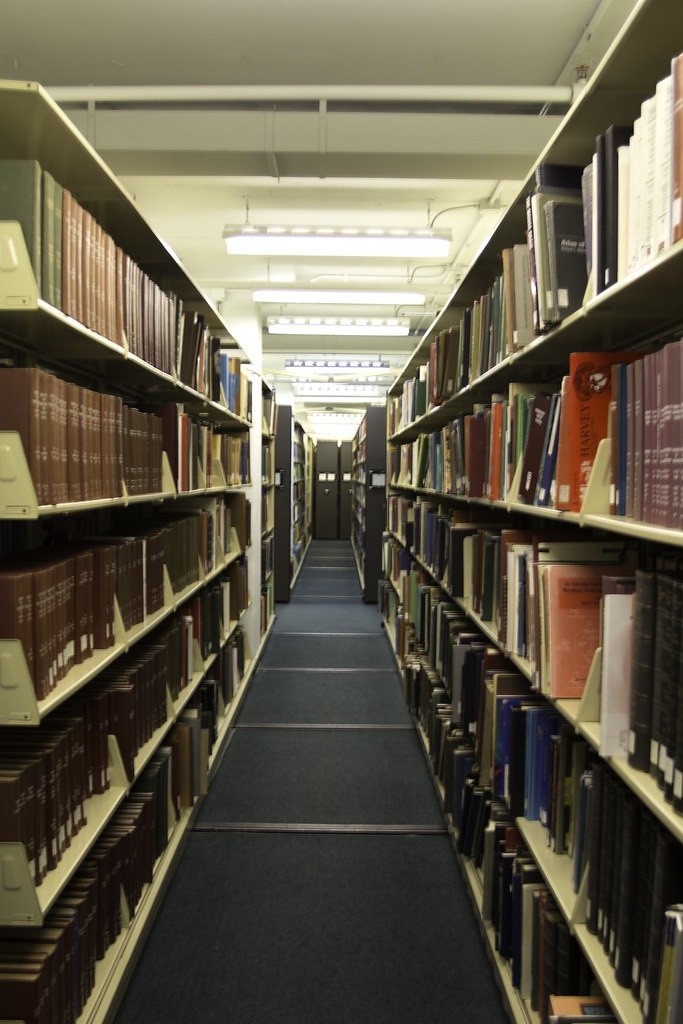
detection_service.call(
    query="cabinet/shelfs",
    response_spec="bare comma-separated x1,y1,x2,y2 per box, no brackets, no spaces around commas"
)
0,80,313,1024
351,2,683,1023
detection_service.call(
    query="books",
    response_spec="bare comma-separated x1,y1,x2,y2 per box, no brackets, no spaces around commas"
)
1,156,312,1024
350,51,683,1023
